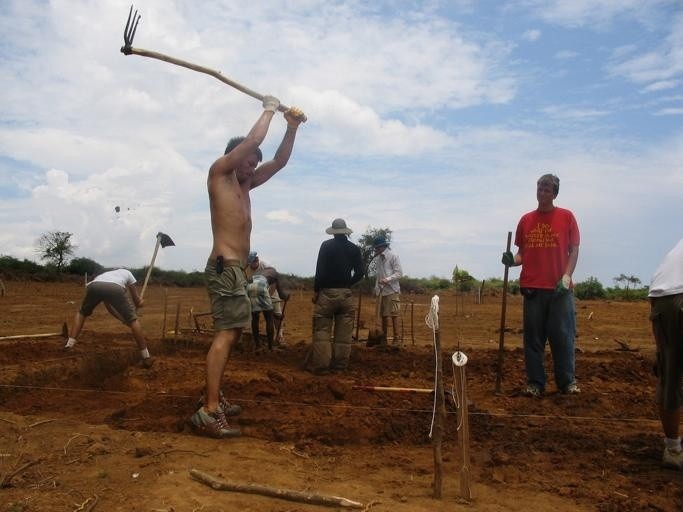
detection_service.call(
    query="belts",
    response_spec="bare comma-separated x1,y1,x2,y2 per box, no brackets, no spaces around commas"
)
208,258,253,281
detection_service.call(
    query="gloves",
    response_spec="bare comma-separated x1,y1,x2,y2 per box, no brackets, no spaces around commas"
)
263,94,279,113
502,252,514,266
283,105,307,129
555,275,570,298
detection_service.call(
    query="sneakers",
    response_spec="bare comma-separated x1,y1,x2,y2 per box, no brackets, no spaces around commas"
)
196,390,241,416
662,446,683,468
192,407,241,437
559,383,581,393
523,385,544,398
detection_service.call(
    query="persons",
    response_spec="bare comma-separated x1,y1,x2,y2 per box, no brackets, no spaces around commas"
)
373,237,403,345
245,252,284,342
190,95,303,439
306,218,364,376
65,269,153,369
648,239,683,470
246,267,289,352
502,174,582,396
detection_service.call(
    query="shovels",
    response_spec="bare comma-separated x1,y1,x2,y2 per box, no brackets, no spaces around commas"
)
367,282,385,346
352,385,474,406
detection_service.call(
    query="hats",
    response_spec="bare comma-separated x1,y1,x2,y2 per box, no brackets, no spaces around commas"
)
326,219,352,234
374,239,386,247
